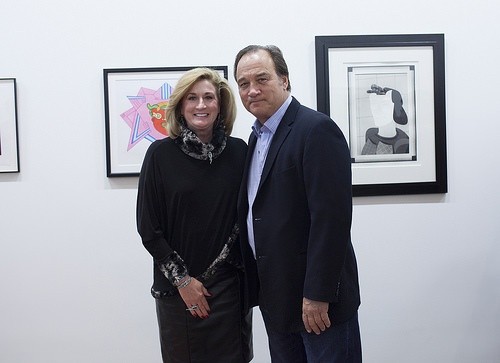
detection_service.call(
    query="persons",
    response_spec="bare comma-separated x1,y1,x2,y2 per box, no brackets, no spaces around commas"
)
233,45,363,363
135,67,261,362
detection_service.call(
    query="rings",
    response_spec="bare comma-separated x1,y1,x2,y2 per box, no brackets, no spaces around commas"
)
190,306,198,311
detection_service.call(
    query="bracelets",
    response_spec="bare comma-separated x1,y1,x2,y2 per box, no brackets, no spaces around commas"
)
175,276,191,289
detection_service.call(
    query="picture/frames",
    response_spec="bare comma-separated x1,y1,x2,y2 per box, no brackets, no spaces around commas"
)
0,78,20,174
315,33,449,198
103,65,229,178
